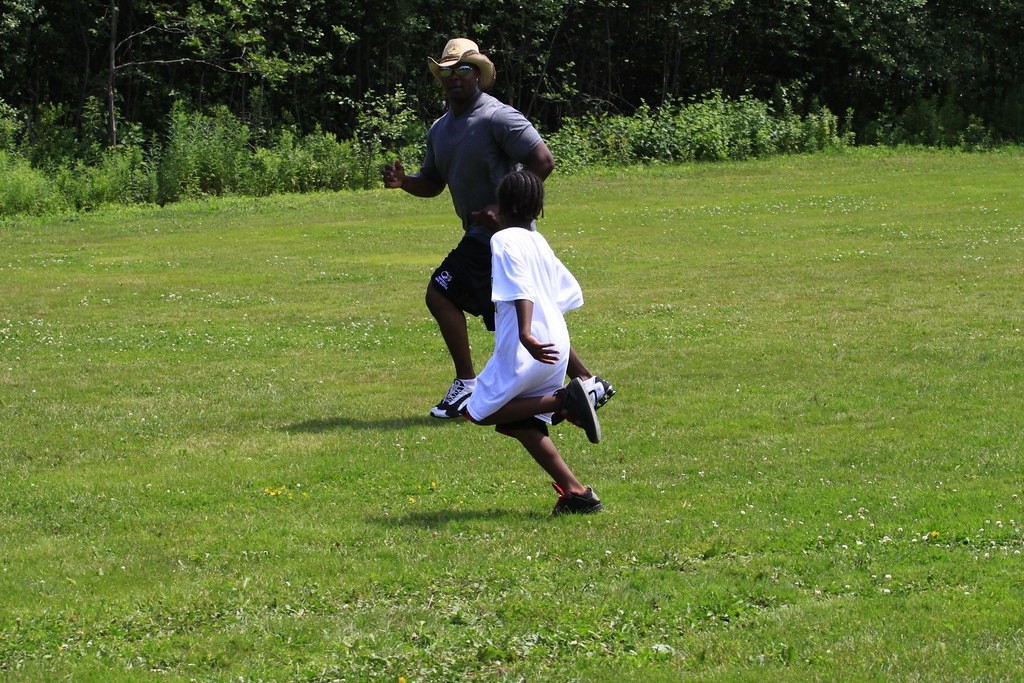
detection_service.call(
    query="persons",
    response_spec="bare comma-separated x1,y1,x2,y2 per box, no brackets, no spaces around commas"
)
464,168,604,526
384,36,617,425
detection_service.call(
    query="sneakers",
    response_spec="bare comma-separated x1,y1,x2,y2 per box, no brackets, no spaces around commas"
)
550,377,602,444
584,376,616,412
429,379,473,418
544,482,603,524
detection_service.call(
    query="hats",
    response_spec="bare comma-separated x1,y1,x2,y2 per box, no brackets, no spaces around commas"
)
426,37,496,93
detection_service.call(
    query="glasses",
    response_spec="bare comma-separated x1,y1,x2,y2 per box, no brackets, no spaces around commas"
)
437,66,481,77
